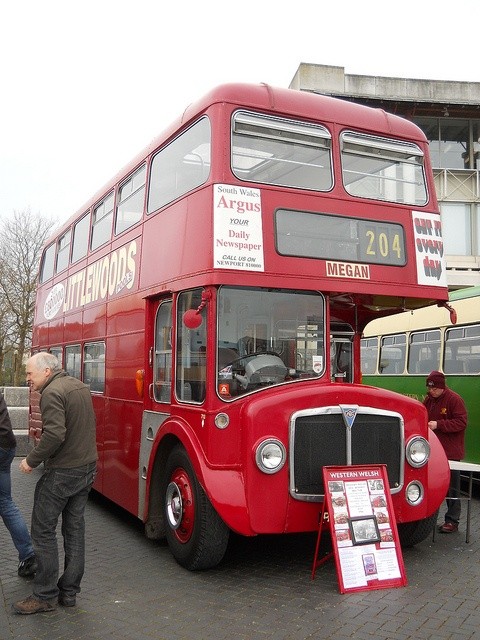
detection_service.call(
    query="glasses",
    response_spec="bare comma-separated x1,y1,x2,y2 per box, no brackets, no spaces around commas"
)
426,386,441,390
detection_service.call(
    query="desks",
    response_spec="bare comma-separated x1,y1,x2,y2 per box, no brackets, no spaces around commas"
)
431,461,480,543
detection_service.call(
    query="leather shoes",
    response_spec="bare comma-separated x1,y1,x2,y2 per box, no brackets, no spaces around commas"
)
439,523,458,533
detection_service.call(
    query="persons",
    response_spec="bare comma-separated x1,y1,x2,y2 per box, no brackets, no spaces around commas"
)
11,351,99,616
422,371,466,534
0,392,39,577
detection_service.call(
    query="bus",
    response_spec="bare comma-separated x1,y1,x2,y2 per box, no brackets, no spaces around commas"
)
29,82,459,572
360,287,480,501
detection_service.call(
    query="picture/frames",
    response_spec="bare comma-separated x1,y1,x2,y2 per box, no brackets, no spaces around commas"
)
347,514,381,547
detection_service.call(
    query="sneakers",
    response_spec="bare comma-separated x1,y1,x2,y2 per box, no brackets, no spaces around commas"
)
18,556,37,577
58,599,76,606
14,593,54,614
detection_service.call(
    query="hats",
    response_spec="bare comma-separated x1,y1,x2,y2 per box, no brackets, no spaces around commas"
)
426,371,445,389
